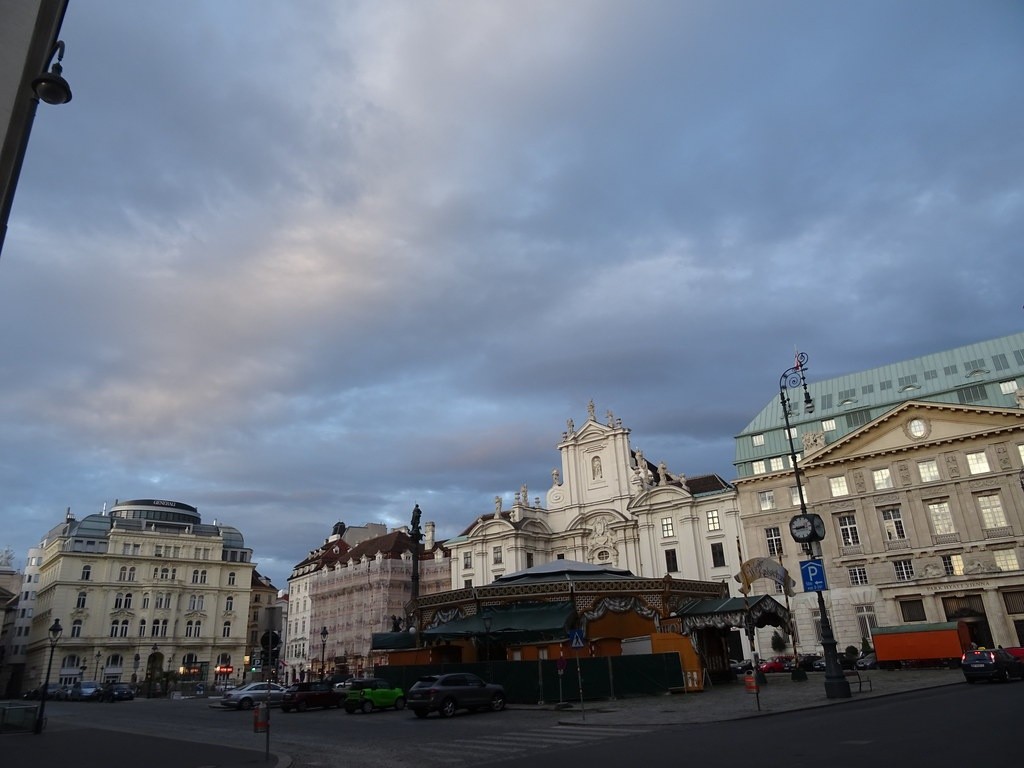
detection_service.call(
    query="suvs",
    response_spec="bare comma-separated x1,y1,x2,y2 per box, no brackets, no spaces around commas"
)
406,673,506,718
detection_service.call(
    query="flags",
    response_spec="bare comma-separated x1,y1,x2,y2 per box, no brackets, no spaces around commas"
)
794,351,801,370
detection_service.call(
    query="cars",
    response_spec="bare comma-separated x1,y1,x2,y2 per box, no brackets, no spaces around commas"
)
856,652,901,670
40,682,68,700
279,682,346,711
70,681,104,703
784,655,821,671
106,682,134,703
779,353,852,698
961,647,1024,684
737,659,764,674
758,656,791,672
342,678,406,713
812,649,859,671
221,682,285,710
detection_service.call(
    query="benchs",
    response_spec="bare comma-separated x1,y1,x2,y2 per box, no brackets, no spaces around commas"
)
843,671,873,693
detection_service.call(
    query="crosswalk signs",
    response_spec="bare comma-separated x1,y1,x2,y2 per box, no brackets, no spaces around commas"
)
571,632,585,648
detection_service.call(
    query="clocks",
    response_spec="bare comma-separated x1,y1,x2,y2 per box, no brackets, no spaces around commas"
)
788,513,826,544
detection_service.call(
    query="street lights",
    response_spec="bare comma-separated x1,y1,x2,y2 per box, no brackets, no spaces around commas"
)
93,650,102,680
1,0,73,252
482,613,495,680
33,618,62,734
319,627,329,680
144,643,158,697
